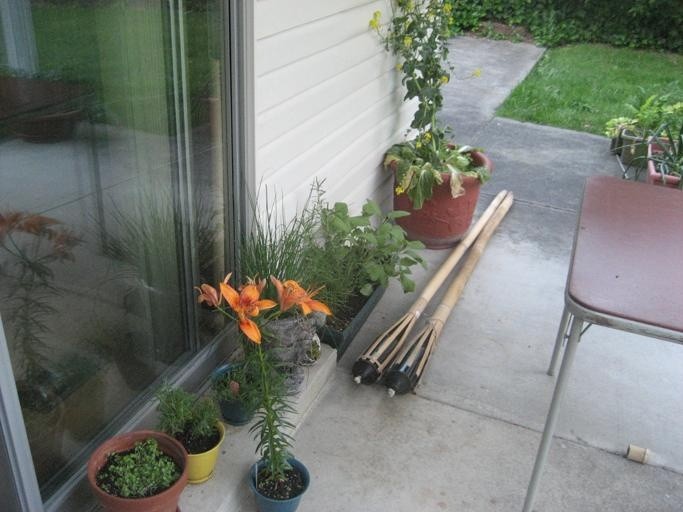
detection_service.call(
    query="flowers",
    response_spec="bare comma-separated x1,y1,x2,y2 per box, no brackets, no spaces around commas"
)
366,0,492,211
6,209,78,407
193,263,335,496
214,364,260,404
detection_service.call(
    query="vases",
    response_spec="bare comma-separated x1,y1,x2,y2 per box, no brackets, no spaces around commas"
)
381,143,492,249
16,389,70,483
213,364,262,424
243,457,310,510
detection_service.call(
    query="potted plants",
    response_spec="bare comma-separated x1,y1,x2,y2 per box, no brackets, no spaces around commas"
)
293,198,431,359
225,175,338,396
602,87,682,188
91,431,191,512
154,376,226,484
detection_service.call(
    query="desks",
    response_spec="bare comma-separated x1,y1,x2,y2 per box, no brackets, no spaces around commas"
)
520,175,682,510
1,71,113,267
